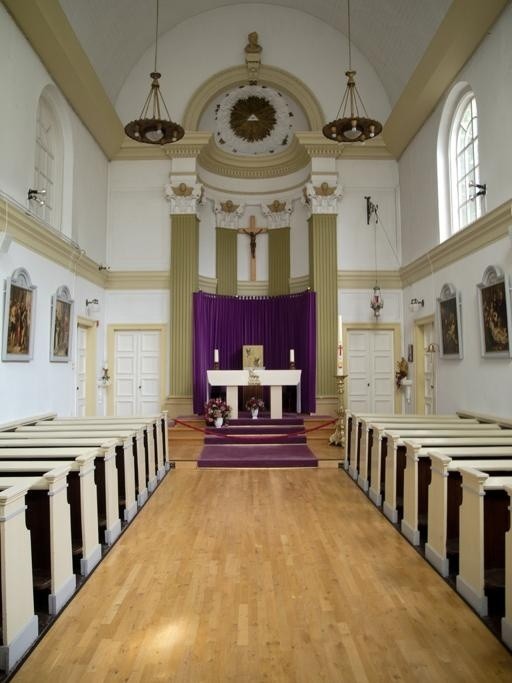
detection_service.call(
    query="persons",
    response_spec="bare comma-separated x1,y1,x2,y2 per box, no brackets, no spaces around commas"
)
243,227,263,258
11,299,28,352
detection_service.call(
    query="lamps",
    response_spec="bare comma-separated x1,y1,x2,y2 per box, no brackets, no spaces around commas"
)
321,0,385,148
122,0,187,149
84,298,101,318
365,194,386,322
98,363,113,387
423,341,440,360
406,295,425,314
26,186,49,211
463,179,488,203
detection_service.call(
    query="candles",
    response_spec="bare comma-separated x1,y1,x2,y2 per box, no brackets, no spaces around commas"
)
288,347,296,362
334,312,346,376
213,348,220,363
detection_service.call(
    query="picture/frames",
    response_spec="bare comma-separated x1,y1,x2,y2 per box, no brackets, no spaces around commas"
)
2,266,41,363
48,282,76,365
475,262,511,361
433,278,465,361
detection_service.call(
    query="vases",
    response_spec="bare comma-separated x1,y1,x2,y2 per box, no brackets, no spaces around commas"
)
249,406,260,420
212,417,224,429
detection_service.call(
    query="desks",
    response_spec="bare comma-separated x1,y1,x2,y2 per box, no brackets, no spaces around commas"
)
204,367,304,420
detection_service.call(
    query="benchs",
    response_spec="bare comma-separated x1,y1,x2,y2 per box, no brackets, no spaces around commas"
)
343,408,512,655
0,410,171,675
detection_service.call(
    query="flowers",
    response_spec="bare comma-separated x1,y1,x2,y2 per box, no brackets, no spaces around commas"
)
203,397,234,426
243,394,266,413
